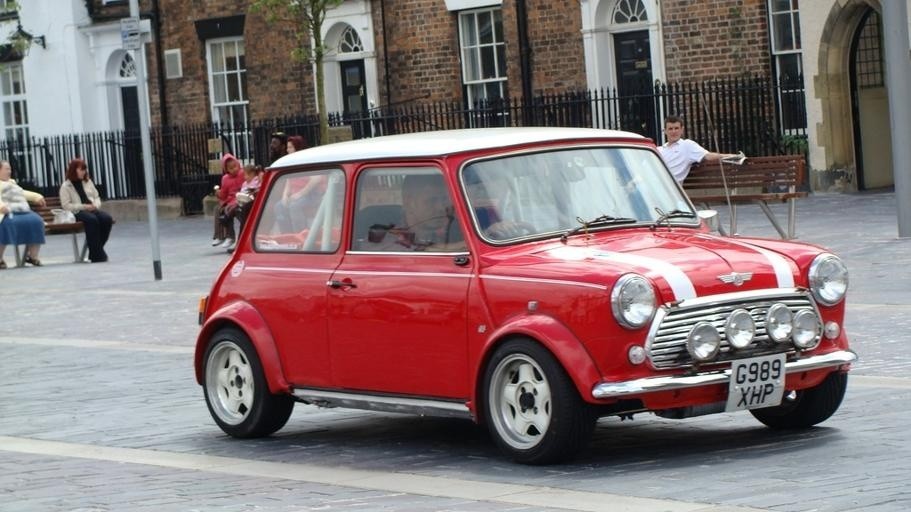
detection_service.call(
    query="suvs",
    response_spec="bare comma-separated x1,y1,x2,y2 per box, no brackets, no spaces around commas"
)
192,125,857,466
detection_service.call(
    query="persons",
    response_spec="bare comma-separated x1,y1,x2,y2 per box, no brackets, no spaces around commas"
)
227,130,288,253
614,112,747,222
375,173,525,254
0,158,48,270
284,136,312,154
211,152,245,247
220,163,265,224
58,158,111,262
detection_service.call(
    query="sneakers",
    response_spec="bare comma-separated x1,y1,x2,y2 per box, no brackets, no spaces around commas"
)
227,243,236,253
221,238,234,248
88,253,108,263
0,261,7,269
212,238,225,246
25,255,42,266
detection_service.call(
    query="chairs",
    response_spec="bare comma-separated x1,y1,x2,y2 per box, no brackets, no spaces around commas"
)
449,207,490,244
352,205,406,248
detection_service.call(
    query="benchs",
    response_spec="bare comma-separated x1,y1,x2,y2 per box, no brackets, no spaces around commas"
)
14,196,116,269
682,153,808,241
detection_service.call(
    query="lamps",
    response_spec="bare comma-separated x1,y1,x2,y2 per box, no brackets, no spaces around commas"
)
10,24,45,57
